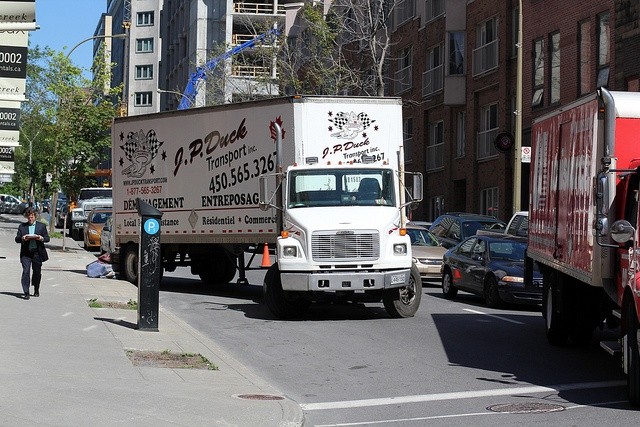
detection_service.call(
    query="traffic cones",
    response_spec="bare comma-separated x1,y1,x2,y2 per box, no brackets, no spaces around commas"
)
261,241,273,267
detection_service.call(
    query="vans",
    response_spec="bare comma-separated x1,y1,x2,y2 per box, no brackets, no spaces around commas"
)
506,210,528,238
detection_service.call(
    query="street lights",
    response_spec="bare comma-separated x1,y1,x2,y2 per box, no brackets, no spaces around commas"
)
49,32,129,229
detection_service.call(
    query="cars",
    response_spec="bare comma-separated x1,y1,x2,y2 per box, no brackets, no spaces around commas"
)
440,233,542,307
57,201,70,227
404,225,448,280
84,208,111,251
406,221,433,230
100,216,112,255
0,194,28,215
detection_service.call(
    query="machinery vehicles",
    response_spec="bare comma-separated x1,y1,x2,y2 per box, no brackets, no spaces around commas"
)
68,168,112,241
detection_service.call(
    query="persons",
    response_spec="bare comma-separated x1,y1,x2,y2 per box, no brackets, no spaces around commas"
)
15,210,50,299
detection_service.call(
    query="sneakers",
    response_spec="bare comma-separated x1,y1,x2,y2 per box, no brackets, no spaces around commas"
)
35,287,39,297
25,295,29,299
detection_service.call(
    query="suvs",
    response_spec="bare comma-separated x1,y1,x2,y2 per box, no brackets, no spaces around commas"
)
428,213,506,249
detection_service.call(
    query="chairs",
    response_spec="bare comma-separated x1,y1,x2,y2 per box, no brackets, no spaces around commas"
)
359,178,381,194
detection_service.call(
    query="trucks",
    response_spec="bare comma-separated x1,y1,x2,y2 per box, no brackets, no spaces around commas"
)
527,87,638,407
112,94,422,319
